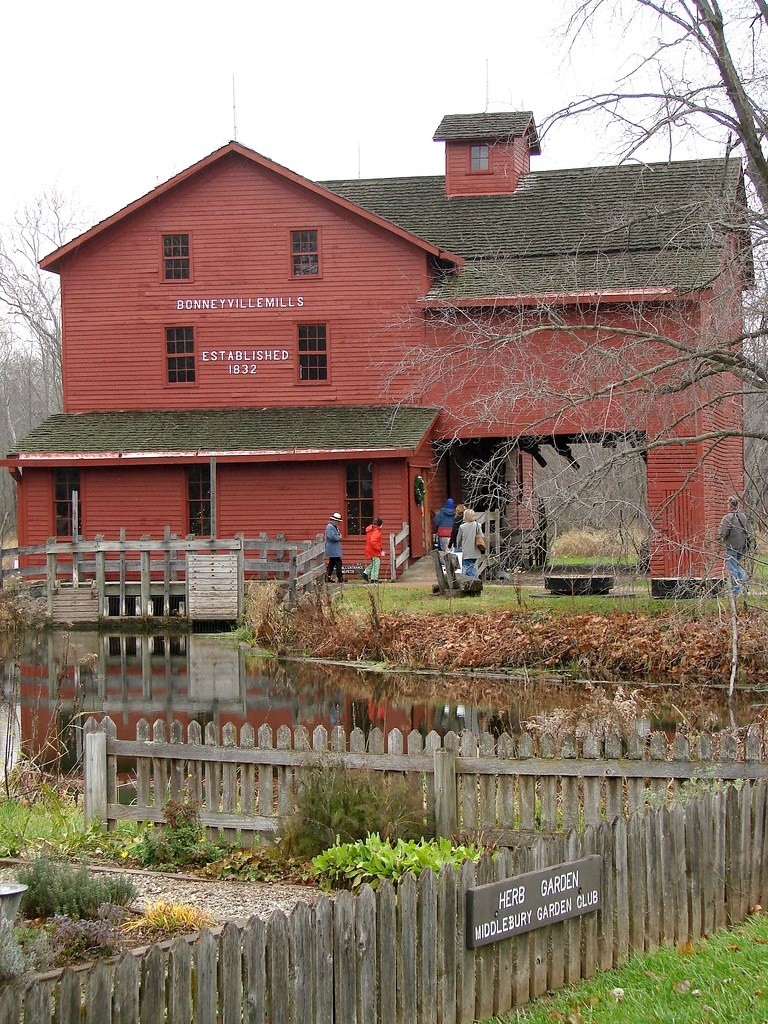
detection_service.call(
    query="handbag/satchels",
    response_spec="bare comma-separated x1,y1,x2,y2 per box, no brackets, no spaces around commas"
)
745,539,750,551
476,523,486,550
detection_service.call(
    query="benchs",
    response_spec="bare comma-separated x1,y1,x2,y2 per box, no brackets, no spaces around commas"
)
432,551,483,600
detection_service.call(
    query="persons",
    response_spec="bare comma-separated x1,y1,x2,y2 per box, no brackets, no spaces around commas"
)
324,513,349,583
434,498,484,577
363,518,385,583
719,497,754,596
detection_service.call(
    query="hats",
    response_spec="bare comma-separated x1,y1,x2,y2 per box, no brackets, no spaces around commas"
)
329,513,343,521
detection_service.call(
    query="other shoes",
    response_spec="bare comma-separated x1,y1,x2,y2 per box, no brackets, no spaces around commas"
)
326,577,335,583
338,578,348,582
371,580,382,583
362,573,369,582
